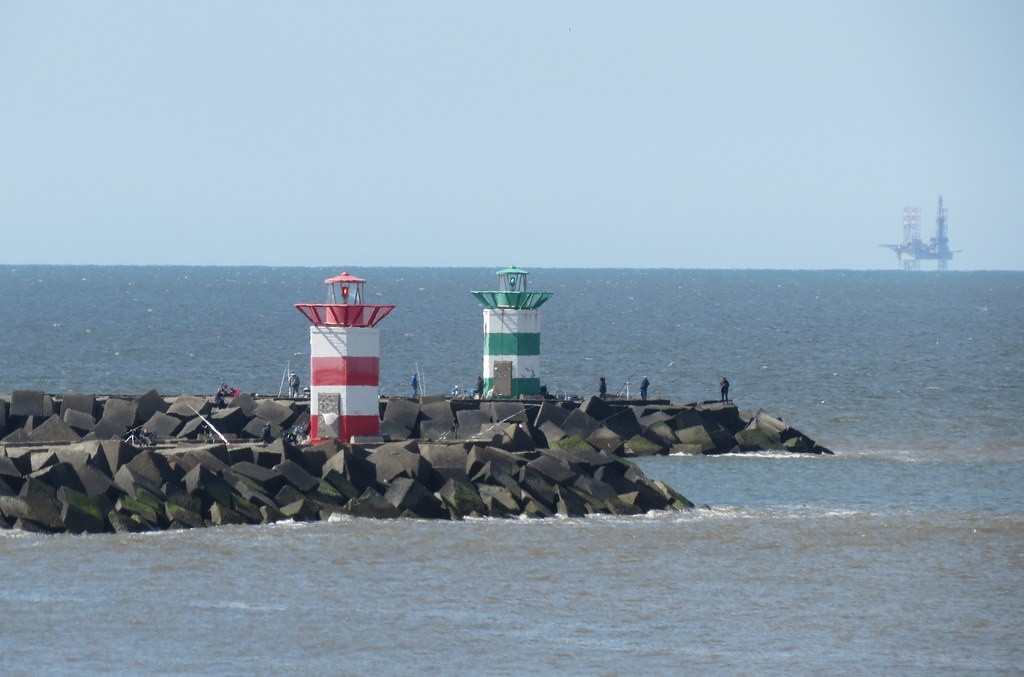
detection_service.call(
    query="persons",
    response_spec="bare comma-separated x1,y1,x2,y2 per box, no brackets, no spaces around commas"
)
599,376,607,399
641,376,650,399
410,374,418,398
288,371,300,396
261,423,272,444
720,377,729,404
215,384,228,409
476,376,484,400
140,428,156,445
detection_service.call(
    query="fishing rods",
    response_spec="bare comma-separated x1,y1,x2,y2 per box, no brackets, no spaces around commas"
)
415,361,424,396
637,358,674,397
712,353,724,391
118,415,163,438
287,361,293,398
611,371,637,401
469,404,541,440
